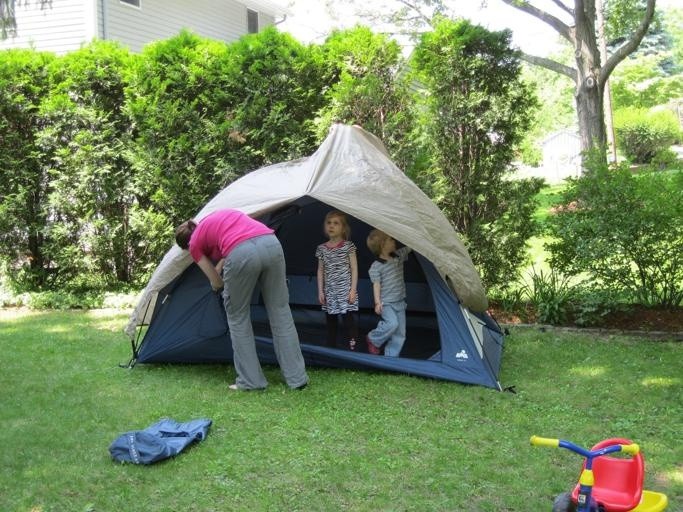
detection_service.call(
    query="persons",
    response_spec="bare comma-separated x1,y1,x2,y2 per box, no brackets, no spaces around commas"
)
174,207,311,390
365,228,412,359
313,209,361,351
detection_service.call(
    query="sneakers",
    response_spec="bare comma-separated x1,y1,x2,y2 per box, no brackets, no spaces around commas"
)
366,335,380,355
347,339,357,353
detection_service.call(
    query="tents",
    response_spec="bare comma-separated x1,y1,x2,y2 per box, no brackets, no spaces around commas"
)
119,122,514,393
